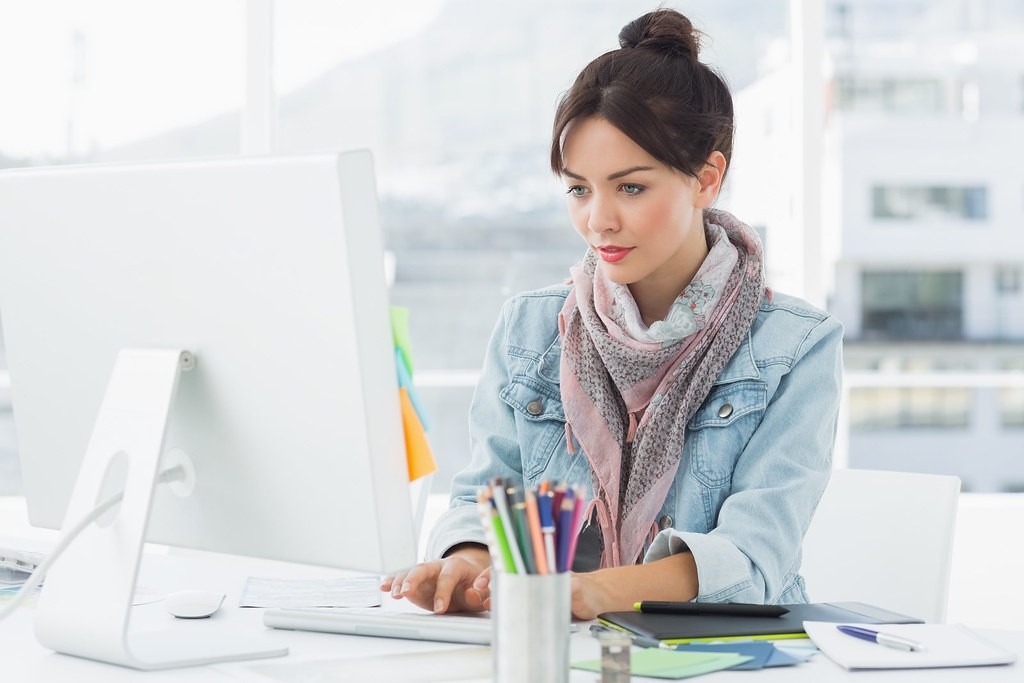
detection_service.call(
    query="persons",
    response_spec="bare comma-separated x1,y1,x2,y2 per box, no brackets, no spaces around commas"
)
380,8,844,620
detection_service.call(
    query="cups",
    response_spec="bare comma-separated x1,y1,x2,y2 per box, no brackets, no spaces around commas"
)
489,567,573,682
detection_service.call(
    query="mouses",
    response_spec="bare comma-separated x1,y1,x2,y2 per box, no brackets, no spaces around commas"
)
168,579,227,617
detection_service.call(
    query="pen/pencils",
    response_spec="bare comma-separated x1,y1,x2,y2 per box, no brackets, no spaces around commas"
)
632,600,791,618
588,623,670,650
475,475,585,575
836,625,925,653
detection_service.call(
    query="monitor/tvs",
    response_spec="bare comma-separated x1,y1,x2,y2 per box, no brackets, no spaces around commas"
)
0,149,420,670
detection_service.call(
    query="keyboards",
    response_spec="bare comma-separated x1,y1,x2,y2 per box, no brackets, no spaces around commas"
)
263,605,493,644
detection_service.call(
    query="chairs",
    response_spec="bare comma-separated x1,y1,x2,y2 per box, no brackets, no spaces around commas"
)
796,463,962,622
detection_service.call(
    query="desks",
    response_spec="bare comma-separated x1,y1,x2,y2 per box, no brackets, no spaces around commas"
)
0,526,1024,683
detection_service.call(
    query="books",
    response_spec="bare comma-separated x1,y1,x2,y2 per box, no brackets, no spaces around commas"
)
802,620,1016,670
